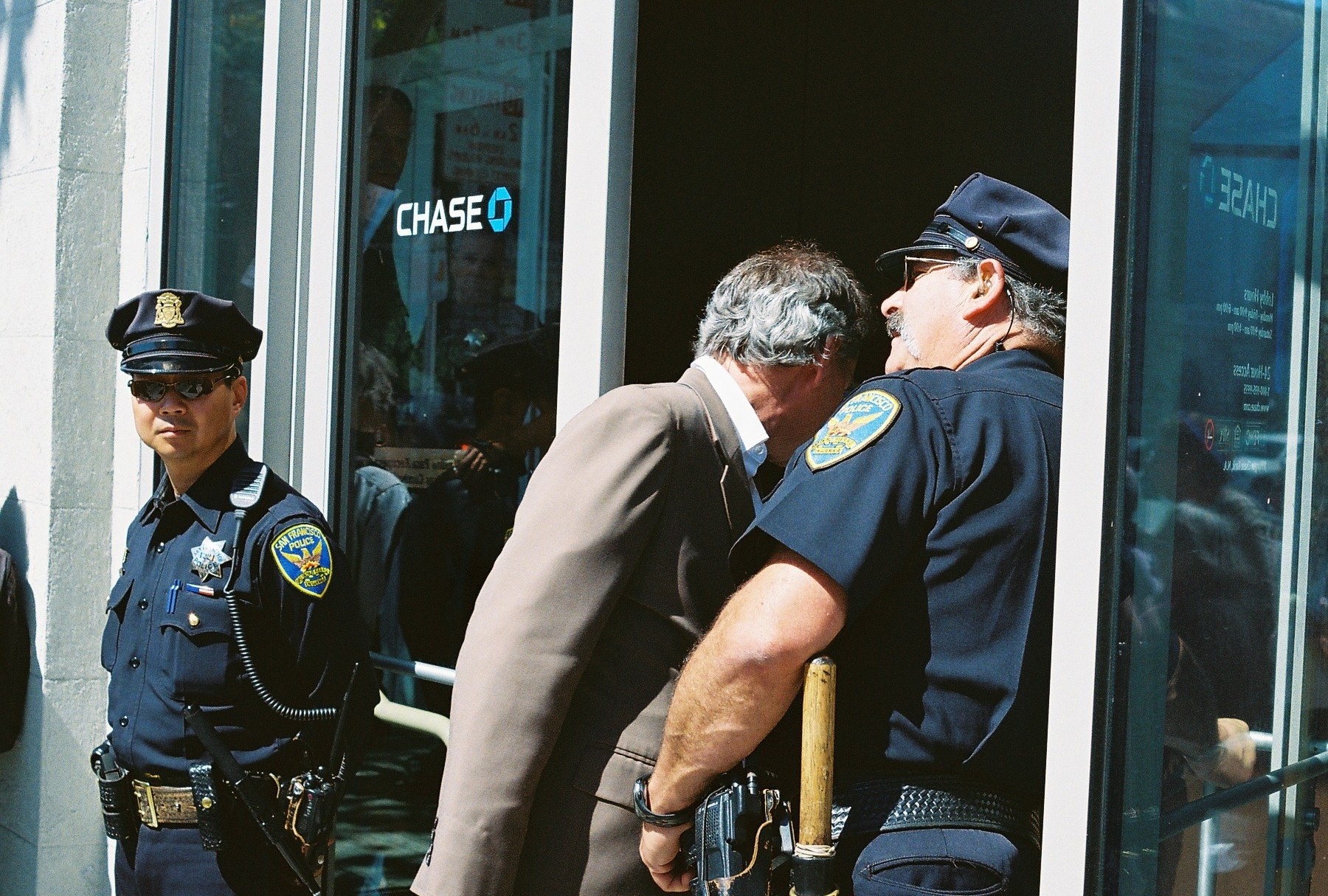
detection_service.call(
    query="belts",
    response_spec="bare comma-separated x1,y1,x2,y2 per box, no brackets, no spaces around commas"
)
799,773,1040,842
129,779,201,829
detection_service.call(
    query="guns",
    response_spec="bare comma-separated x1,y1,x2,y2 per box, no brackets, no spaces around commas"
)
98,755,139,871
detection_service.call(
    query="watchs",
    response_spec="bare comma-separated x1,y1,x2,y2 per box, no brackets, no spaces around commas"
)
633,773,695,827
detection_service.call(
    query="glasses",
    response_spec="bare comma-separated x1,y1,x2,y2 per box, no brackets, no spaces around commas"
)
127,376,234,403
903,255,1012,294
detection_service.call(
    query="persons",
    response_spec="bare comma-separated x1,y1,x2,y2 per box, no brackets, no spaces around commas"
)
100,288,389,896
408,237,875,896
394,219,541,436
384,298,556,717
0,547,31,753
1125,417,1270,896
640,171,1257,896
344,340,416,713
360,86,412,336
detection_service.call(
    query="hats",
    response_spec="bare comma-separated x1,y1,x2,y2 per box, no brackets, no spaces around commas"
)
106,289,262,375
876,172,1069,300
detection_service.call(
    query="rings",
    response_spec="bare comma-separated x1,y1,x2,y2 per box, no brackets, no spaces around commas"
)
477,469,480,472
